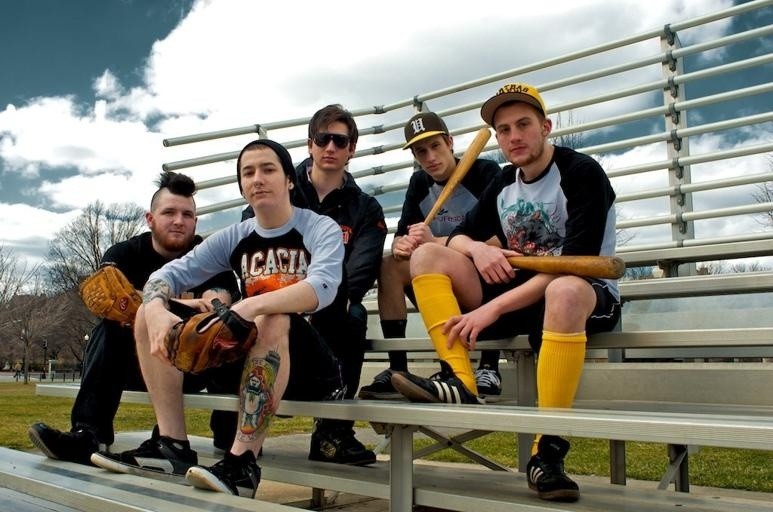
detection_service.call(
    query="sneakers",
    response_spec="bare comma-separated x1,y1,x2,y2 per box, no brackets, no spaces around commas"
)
390,358,486,405
211,435,263,458
185,448,262,499
473,365,503,403
91,424,198,486
525,433,581,500
28,421,108,467
308,424,376,465
359,367,409,400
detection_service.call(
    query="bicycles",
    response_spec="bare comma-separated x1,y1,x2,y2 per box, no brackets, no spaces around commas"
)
12,369,22,379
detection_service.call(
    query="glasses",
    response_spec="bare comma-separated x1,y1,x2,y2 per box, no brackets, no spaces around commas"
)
311,132,352,148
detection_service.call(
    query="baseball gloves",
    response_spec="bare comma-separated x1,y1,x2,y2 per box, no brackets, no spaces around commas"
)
160,298,257,376
78,263,140,327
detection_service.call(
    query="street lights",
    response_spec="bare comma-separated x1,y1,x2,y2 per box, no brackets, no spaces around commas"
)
84,332,89,354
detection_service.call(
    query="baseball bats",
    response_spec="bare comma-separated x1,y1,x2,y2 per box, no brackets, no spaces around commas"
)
505,255,627,279
399,128,491,262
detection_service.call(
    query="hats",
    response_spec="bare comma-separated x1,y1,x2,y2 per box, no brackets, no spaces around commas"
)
236,139,297,195
402,111,449,151
481,82,548,131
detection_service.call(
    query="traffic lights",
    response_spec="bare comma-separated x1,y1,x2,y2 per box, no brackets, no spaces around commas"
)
42,335,48,349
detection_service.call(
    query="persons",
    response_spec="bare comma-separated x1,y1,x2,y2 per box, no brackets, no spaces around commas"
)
88,138,346,500
239,103,388,467
389,81,623,503
356,111,506,404
27,170,265,467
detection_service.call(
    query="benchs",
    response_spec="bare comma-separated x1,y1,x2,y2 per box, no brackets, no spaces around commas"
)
0,234,772,512
